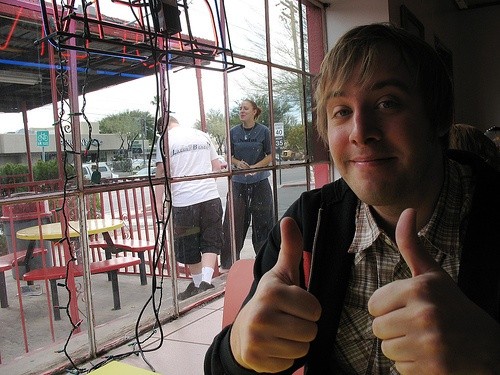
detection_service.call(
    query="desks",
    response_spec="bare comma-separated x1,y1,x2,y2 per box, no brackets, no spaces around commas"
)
16,218,121,266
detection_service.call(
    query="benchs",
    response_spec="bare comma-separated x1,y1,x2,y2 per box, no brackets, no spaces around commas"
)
0,247,47,308
23,256,141,321
89,239,160,286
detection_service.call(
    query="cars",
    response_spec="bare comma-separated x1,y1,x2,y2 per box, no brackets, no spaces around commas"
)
150,158,156,167
130,159,146,171
127,167,157,178
72,162,119,183
275,153,282,161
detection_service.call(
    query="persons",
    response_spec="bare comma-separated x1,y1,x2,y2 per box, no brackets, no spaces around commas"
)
155,117,222,299
204,23,499,375
450,122,499,163
219,100,274,270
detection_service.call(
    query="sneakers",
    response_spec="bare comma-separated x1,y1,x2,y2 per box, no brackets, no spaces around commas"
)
176,280,216,299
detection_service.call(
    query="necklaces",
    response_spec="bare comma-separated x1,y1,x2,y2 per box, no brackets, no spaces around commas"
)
243,122,255,140
